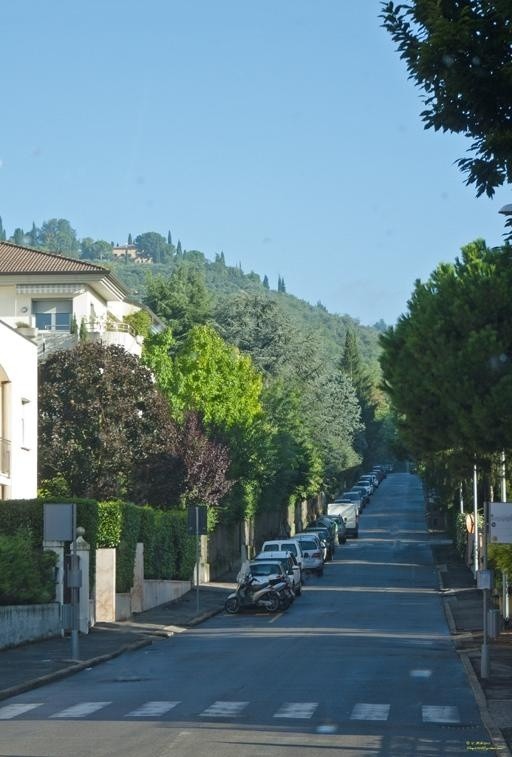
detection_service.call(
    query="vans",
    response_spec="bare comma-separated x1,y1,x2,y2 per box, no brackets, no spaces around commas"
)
291,532,326,578
261,539,305,573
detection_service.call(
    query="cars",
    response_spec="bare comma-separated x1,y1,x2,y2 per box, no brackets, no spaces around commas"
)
334,464,386,514
237,562,296,604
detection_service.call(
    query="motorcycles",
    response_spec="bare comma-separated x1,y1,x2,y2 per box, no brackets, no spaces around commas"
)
253,573,297,611
223,572,288,614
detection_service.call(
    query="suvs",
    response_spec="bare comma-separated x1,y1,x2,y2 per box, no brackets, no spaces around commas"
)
301,501,358,564
254,550,303,597
